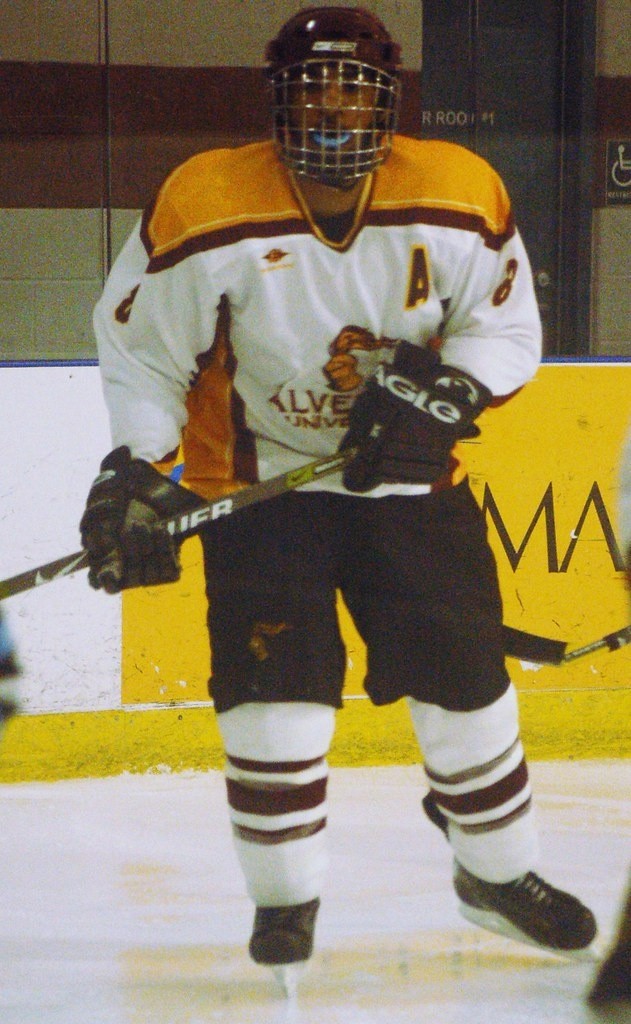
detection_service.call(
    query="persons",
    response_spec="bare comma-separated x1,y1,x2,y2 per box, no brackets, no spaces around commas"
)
79,8,604,995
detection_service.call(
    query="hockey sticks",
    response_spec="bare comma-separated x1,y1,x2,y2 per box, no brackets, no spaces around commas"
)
503,624,631,667
0,442,358,601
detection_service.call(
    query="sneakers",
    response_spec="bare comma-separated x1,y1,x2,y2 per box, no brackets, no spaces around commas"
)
453,855,598,964
250,896,320,1000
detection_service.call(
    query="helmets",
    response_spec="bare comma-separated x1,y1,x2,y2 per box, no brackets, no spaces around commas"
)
264,7,401,184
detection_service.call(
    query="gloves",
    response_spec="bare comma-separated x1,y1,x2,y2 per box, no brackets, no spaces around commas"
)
337,338,491,494
80,445,210,594
